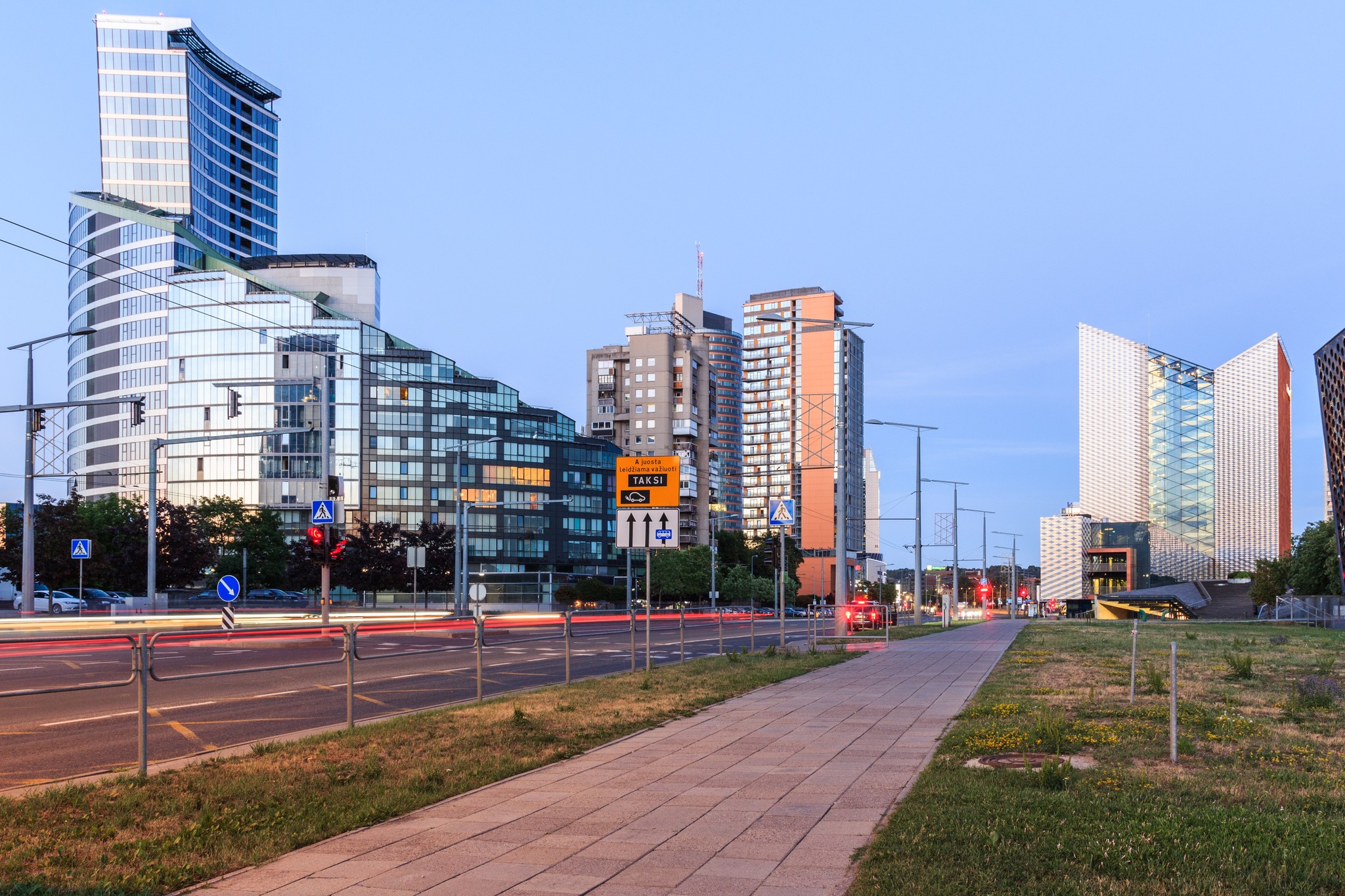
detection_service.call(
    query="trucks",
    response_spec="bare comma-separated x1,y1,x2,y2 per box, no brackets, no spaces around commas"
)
0,568,49,601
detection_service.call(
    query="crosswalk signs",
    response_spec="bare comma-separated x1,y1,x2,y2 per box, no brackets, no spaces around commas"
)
311,499,334,524
71,540,91,560
769,499,796,525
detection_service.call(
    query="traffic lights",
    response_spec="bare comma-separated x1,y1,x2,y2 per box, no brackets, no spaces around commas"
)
228,390,243,418
327,475,341,498
33,408,46,431
308,528,326,565
328,527,347,561
132,401,146,425
764,537,778,567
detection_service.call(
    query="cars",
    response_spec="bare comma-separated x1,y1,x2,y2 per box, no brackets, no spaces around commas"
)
13,590,87,612
95,592,134,604
286,591,310,608
576,603,834,621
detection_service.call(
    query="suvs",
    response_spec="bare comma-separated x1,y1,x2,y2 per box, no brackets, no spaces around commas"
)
188,589,240,611
847,599,898,630
58,588,122,613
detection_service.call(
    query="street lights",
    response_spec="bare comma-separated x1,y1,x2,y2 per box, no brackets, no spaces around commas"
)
442,437,503,621
812,566,816,600
849,419,1023,624
706,514,740,613
750,554,758,607
811,575,814,595
8,326,97,618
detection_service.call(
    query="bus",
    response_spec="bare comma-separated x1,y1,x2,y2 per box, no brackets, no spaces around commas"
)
245,589,299,608
